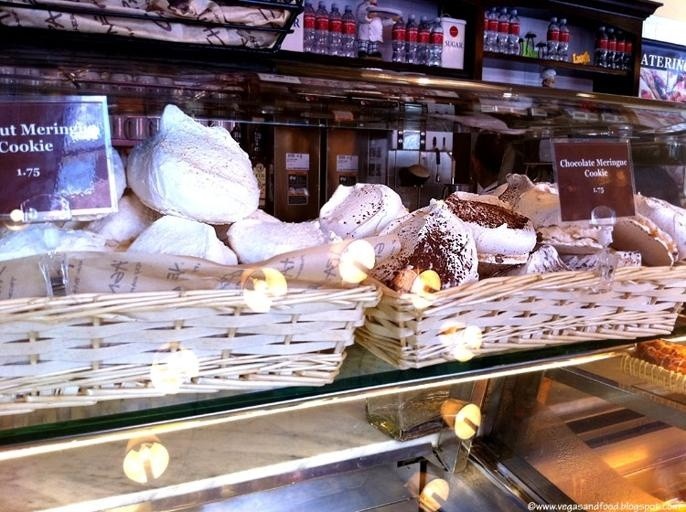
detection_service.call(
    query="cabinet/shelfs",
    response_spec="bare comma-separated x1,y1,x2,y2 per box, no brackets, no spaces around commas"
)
303,0,663,98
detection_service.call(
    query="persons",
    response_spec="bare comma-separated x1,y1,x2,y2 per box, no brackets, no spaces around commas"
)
355,0,400,59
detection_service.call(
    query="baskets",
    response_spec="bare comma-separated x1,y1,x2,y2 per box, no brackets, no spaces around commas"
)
0,265,686,417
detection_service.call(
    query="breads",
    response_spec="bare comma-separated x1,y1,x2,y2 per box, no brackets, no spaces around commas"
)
0,104,686,293
634,338,686,374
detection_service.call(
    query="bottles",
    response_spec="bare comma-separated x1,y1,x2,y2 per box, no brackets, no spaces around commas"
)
391,14,444,68
593,24,633,71
303,0,358,58
483,8,521,56
546,16,570,62
